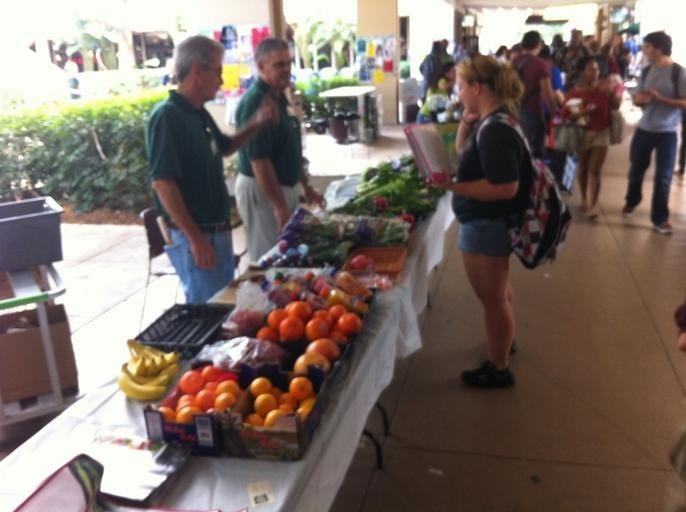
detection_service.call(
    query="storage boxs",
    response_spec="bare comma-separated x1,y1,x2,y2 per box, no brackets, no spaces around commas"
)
0,266,54,305
0,303,79,405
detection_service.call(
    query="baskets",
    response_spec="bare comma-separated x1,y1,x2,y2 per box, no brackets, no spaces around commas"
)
132,302,237,357
340,245,408,282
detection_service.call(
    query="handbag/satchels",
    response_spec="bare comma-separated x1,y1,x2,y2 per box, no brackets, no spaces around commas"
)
611,108,625,144
543,121,586,153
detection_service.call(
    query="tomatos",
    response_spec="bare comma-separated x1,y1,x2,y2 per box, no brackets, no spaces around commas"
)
351,254,369,269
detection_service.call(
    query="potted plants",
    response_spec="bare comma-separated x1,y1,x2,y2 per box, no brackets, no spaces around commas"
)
294,79,361,142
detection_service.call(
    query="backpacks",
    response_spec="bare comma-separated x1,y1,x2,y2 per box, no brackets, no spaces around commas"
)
473,113,571,270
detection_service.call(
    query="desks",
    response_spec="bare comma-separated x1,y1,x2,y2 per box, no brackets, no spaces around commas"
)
230,172,460,438
0,257,402,512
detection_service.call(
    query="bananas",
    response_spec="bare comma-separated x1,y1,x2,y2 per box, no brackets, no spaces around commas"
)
118,340,181,401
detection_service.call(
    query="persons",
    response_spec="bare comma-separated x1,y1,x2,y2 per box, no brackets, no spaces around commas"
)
143,34,279,306
230,38,327,269
418,26,684,234
432,55,539,389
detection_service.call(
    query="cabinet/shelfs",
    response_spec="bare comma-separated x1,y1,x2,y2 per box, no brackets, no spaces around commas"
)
0,262,85,433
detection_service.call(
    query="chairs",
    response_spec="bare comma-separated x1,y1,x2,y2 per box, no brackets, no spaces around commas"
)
138,205,241,327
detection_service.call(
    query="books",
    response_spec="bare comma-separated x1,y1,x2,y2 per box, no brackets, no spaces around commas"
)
402,122,454,188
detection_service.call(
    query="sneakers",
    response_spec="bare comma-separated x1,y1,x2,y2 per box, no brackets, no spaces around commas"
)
649,215,673,234
577,199,599,220
458,358,516,389
622,194,642,219
